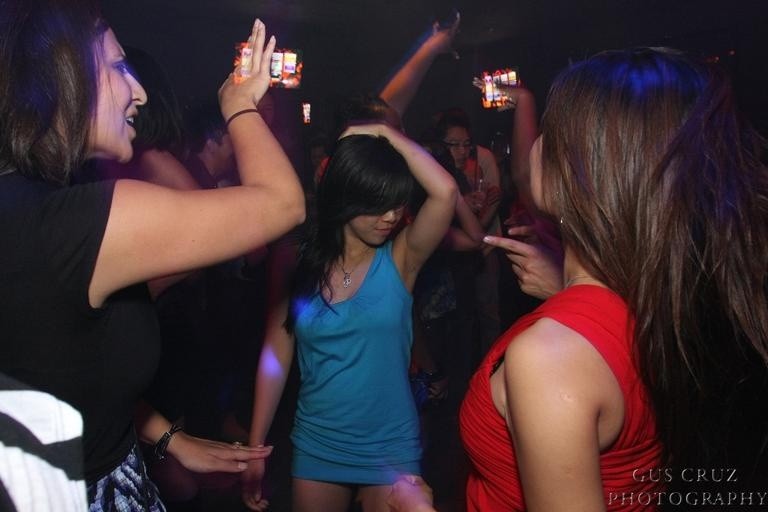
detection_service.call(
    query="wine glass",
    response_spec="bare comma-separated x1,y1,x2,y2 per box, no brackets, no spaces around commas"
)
474,179,487,208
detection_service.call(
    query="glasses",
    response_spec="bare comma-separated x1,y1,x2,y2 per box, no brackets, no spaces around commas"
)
443,138,470,150
